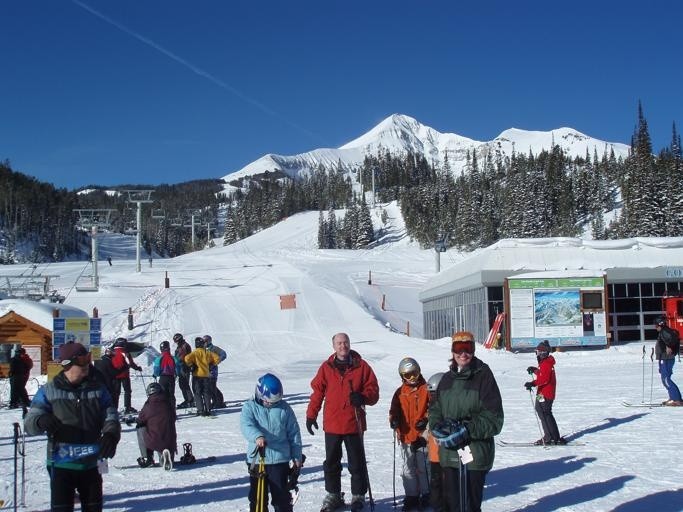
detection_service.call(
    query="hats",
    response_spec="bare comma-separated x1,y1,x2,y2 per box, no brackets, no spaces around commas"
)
59,343,88,370
656,318,667,327
536,339,551,352
452,332,475,344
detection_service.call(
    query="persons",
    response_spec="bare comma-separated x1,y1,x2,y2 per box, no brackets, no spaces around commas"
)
525,340,560,446
306,332,379,512
6,334,226,512
241,373,304,512
389,332,504,512
655,317,683,407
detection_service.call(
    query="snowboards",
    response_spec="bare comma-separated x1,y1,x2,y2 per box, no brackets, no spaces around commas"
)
113,443,216,469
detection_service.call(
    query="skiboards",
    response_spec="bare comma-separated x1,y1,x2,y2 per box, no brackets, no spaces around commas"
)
496,440,585,448
116,400,244,426
317,492,375,512
286,491,300,505
621,400,683,407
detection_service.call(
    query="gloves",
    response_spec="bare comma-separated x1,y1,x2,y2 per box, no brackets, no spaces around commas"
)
524,380,536,388
414,417,429,431
349,391,365,407
390,416,401,429
95,433,118,460
527,366,538,375
430,419,470,450
306,419,319,436
37,412,65,436
136,365,141,370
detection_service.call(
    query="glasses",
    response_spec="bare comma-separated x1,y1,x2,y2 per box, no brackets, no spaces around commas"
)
452,341,475,354
401,369,420,380
67,351,92,367
535,350,546,355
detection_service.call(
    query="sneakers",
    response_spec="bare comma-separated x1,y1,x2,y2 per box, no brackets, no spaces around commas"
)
162,448,173,472
320,493,344,512
534,437,566,446
123,407,135,414
176,400,226,415
662,399,683,407
137,456,155,468
401,493,432,512
350,495,365,512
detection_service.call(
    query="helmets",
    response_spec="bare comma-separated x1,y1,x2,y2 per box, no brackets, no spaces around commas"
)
398,357,421,386
195,337,204,348
160,341,170,352
427,373,446,392
146,382,165,396
173,333,184,344
202,335,212,348
254,373,284,409
116,338,127,347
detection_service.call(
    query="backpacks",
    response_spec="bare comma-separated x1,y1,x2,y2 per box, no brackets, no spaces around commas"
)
659,328,681,356
